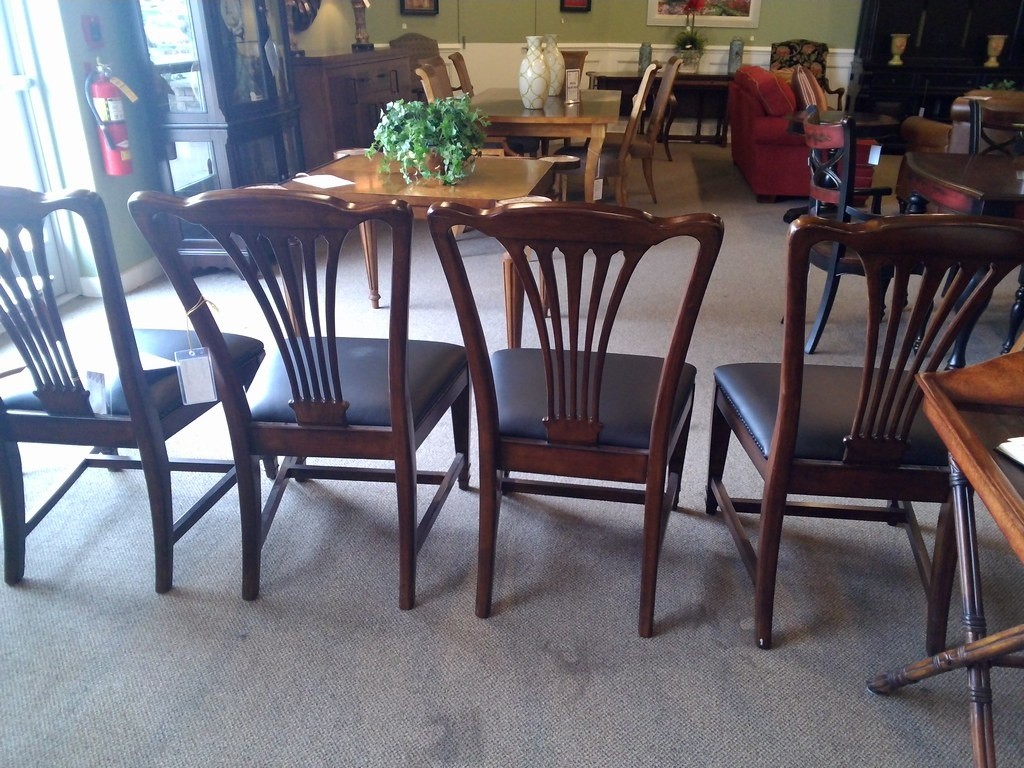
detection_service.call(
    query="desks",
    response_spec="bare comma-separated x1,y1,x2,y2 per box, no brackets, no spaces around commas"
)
866,328,1024,768
783,111,899,223
290,47,411,174
895,151,1024,371
585,71,730,148
271,152,555,349
464,86,622,204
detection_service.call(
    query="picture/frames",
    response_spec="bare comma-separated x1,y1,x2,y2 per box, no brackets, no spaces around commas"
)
400,0,439,14
560,0,591,11
646,0,760,28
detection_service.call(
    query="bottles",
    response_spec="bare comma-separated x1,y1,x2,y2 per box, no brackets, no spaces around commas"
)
543,34,565,96
728,36,743,75
637,41,652,76
519,36,551,109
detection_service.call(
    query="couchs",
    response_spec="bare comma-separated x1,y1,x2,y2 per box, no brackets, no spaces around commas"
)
897,91,1024,220
729,65,879,205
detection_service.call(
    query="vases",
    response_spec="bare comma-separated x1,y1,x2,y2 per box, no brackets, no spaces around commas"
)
674,50,704,72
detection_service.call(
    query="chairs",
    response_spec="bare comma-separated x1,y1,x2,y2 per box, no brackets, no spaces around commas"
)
0,32,1024,649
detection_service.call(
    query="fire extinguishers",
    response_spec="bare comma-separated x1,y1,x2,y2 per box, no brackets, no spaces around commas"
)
85,57,132,175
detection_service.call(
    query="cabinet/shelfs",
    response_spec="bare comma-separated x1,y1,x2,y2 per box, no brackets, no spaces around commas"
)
125,0,305,284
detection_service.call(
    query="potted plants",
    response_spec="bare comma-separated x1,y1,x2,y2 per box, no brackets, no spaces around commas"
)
364,93,492,186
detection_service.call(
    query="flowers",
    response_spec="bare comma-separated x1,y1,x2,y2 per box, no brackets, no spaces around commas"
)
673,0,710,65
